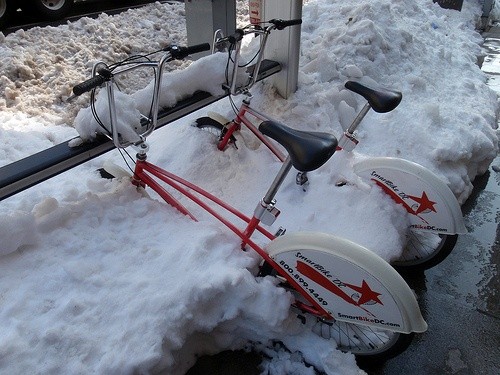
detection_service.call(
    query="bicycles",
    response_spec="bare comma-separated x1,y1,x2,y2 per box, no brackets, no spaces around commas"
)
62,14,463,366
